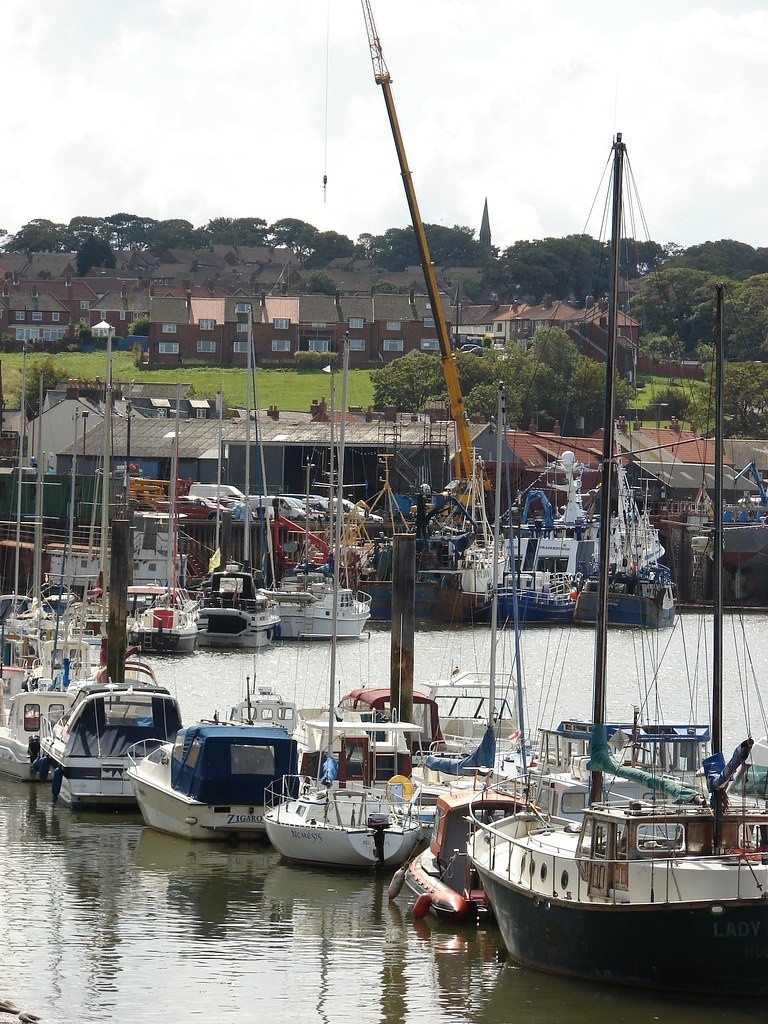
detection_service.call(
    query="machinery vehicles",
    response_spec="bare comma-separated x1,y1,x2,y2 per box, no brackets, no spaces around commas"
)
361,0,493,527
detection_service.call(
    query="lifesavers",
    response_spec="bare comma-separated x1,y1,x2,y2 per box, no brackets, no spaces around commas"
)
385,775,413,806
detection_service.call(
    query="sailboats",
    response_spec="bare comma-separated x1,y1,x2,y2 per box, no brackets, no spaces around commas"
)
0,309,679,922
471,132,768,1010
